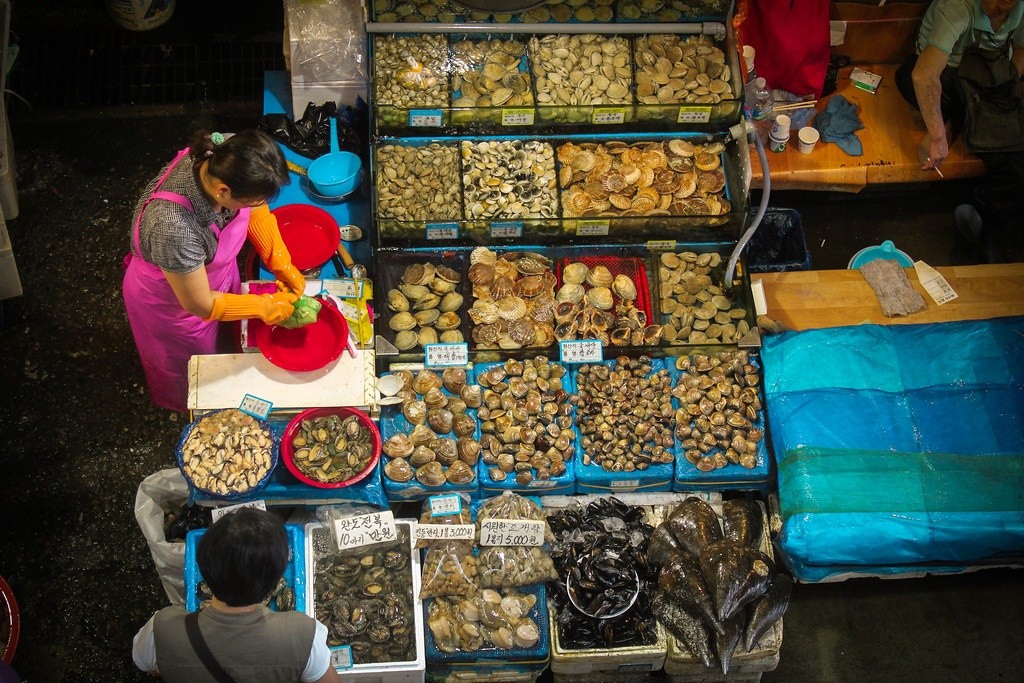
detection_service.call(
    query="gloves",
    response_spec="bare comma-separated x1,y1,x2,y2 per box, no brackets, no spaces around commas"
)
203,291,298,325
247,199,306,298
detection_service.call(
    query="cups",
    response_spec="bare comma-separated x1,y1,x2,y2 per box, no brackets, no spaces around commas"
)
798,126,819,154
769,115,791,143
741,45,756,73
743,70,756,99
767,132,790,152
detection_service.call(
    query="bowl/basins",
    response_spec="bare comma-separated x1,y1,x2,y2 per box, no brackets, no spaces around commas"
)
177,411,278,500
280,407,381,489
848,239,916,272
307,166,366,204
566,551,639,619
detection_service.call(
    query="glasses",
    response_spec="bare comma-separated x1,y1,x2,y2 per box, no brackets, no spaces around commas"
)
230,195,267,206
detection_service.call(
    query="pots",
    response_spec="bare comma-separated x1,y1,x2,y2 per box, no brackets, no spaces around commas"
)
307,116,362,197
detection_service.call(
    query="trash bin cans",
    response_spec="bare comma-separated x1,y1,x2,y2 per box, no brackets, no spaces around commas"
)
746,207,810,270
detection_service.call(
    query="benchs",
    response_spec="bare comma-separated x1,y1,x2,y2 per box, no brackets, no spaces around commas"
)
747,59,984,193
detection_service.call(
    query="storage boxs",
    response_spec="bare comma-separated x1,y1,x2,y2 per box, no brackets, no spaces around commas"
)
543,492,668,683
306,516,426,683
420,497,551,683
667,505,784,683
183,522,304,612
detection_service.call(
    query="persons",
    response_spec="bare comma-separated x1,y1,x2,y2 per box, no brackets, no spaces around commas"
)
898,0,1024,171
120,125,307,416
133,506,340,683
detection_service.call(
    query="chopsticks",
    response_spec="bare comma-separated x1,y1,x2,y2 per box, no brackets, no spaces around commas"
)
774,99,818,112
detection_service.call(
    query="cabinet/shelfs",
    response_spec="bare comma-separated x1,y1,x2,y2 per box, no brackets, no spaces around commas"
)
365,0,763,502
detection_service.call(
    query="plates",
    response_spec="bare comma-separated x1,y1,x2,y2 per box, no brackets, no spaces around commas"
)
254,299,348,373
270,204,340,271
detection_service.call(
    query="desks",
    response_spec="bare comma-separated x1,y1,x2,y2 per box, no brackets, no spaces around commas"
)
754,261,1024,582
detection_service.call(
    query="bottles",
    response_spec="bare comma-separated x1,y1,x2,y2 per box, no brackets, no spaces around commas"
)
747,77,773,152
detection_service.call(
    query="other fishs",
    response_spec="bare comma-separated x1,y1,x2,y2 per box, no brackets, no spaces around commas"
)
546,496,791,676
180,409,276,495
294,414,372,484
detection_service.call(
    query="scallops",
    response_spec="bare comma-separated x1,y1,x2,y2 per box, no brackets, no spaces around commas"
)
470,245,556,348
562,140,732,225
384,368,483,487
452,38,533,122
387,263,466,352
